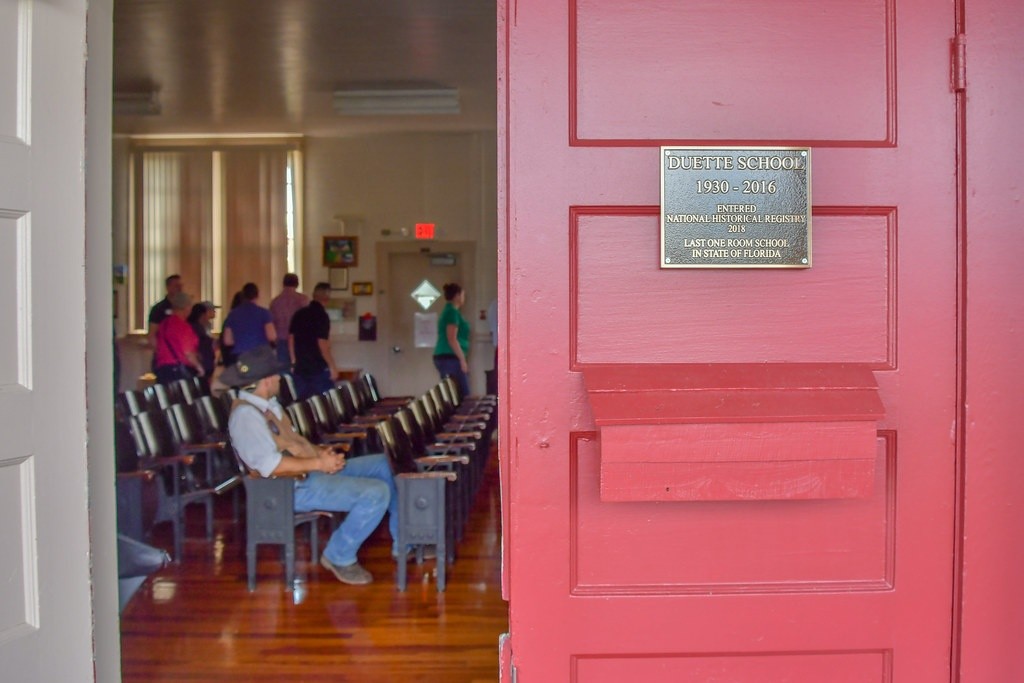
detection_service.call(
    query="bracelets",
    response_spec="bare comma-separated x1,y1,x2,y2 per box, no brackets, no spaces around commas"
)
290,363,295,368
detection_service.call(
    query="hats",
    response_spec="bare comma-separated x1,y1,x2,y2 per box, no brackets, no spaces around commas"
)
202,301,222,309
219,345,296,386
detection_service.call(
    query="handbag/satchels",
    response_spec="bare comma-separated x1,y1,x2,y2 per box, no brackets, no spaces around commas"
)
170,364,193,383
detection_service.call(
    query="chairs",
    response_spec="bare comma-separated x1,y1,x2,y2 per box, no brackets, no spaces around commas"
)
113,364,495,594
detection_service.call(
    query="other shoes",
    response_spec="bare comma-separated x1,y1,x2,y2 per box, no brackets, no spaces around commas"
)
320,553,373,584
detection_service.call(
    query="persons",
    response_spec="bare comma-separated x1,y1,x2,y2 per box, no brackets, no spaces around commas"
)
216,346,436,584
269,273,309,376
288,282,339,402
432,283,472,396
148,274,279,384
487,299,498,385
